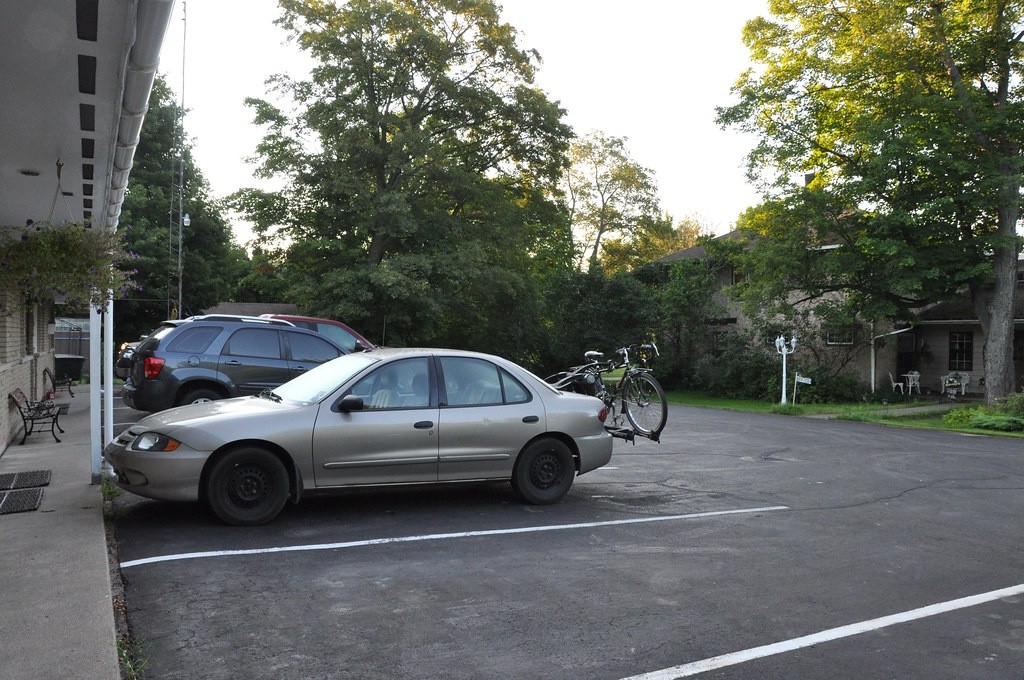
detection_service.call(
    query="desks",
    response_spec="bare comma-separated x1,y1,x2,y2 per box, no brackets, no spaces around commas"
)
901,374,921,395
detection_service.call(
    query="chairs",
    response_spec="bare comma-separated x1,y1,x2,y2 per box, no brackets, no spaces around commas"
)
905,371,922,394
889,373,905,394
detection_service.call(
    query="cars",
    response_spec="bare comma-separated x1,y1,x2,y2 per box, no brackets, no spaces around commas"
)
102,348,614,527
117,342,140,379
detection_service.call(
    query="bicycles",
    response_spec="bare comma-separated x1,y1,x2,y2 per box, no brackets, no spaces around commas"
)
546,343,667,441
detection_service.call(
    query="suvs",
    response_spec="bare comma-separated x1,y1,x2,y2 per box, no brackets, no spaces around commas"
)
258,314,380,352
123,312,352,415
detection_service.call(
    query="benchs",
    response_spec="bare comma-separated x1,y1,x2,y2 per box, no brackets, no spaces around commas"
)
44,366,75,399
941,372,970,395
9,386,64,446
